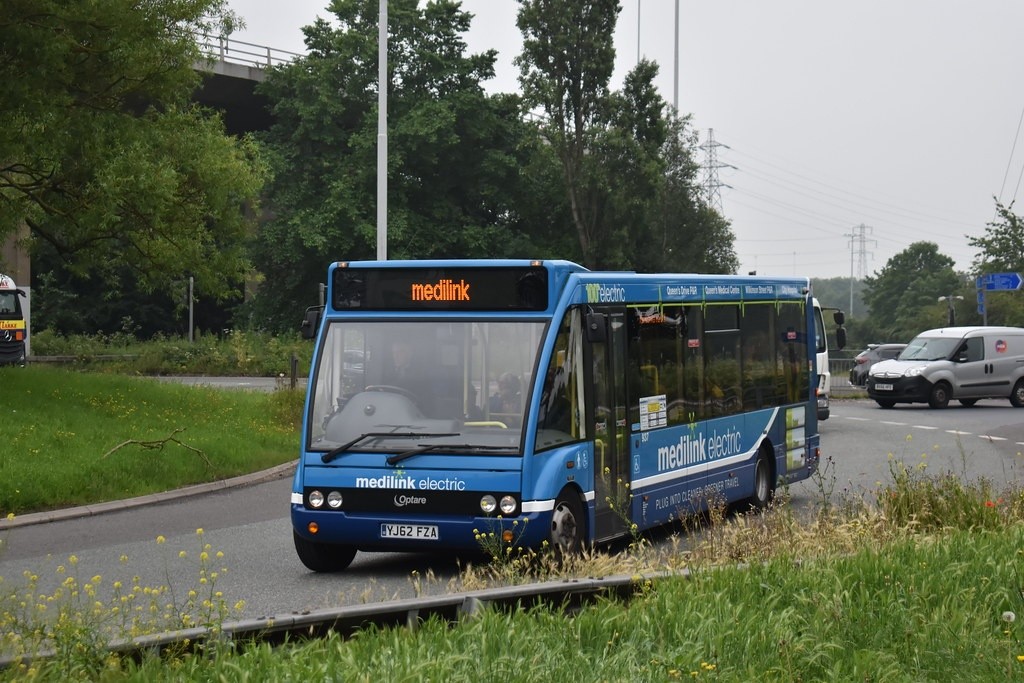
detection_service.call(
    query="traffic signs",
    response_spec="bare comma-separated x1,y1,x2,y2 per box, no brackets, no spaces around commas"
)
982,272,1023,291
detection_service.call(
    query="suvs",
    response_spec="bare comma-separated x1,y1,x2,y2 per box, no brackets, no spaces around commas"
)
849,343,908,390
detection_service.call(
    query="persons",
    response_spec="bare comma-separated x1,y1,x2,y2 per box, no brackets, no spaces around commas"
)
365,339,437,417
451,384,483,421
487,373,521,427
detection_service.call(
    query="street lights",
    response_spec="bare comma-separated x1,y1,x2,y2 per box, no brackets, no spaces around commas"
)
938,295,964,326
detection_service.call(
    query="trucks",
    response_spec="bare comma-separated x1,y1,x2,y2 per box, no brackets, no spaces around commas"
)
0,273,27,368
741,298,848,422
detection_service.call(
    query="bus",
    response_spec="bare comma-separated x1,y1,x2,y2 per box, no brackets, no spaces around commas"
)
288,257,823,579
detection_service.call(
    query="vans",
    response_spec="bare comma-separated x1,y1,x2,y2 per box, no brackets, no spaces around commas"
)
866,326,1024,410
